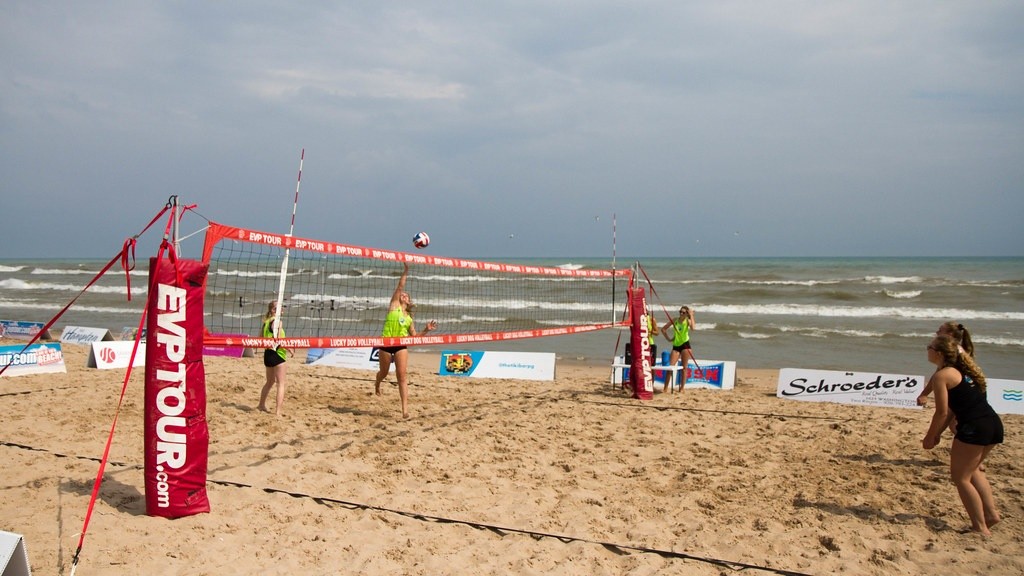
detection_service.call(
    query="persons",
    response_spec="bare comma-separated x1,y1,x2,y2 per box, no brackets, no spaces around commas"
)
662,306,694,392
645,304,660,384
376,262,438,418
258,301,294,413
918,322,1004,534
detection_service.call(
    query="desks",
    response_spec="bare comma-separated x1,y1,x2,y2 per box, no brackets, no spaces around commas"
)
611,364,683,394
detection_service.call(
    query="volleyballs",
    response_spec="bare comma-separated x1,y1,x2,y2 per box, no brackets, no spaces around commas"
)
412,231,431,251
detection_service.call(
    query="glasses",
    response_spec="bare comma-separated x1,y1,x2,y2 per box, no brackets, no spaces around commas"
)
679,310,686,314
927,344,938,351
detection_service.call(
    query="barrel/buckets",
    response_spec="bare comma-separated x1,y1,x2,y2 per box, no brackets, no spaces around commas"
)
625,343,656,382
